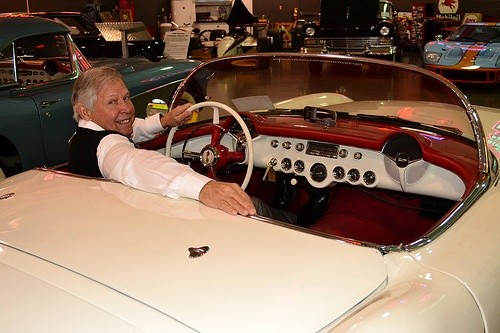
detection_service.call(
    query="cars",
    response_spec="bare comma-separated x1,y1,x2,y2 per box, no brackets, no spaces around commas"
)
189,27,258,60
301,0,409,75
422,21,500,91
0,52,500,333
26,12,165,63
0,13,204,179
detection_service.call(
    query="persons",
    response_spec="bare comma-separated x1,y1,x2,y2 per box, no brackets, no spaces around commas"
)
67,66,328,227
